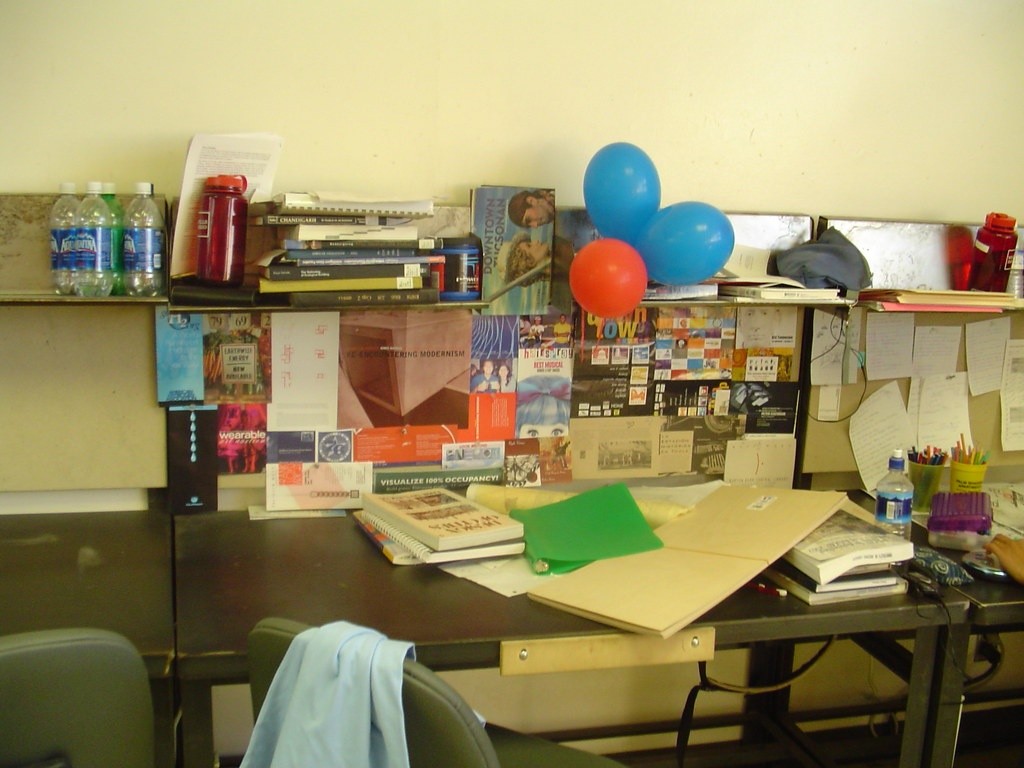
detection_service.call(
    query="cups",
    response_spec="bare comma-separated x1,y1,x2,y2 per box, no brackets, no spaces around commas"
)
908,460,944,511
951,459,987,492
197,175,248,284
966,213,1017,293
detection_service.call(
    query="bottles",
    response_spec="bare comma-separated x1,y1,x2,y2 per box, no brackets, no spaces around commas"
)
875,448,914,543
72,183,112,298
123,184,164,296
50,183,81,295
101,183,123,294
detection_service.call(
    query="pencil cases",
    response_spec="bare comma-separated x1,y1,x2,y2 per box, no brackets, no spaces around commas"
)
910,546,975,586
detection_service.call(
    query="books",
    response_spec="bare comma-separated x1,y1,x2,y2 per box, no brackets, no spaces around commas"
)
170,190,442,312
761,505,916,606
526,483,851,641
640,275,1024,318
352,487,526,567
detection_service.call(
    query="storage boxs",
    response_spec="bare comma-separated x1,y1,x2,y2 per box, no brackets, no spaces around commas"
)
927,491,994,553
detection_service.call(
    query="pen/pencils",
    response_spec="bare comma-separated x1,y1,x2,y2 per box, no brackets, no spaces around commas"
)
908,445,949,466
748,581,787,597
951,432,990,465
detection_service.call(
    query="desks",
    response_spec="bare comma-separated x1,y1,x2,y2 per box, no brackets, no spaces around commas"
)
0,511,178,768
770,491,1024,768
175,489,971,768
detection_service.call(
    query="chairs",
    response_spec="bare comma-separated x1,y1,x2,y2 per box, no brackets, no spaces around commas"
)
247,616,625,767
0,627,154,768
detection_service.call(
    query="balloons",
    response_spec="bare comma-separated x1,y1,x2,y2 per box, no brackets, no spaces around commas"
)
632,200,735,287
583,141,660,245
570,238,647,320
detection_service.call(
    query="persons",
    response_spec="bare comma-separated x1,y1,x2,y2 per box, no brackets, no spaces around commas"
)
983,533,1024,587
737,305,783,349
518,313,571,351
502,231,551,290
515,375,571,440
506,188,555,228
472,359,515,395
